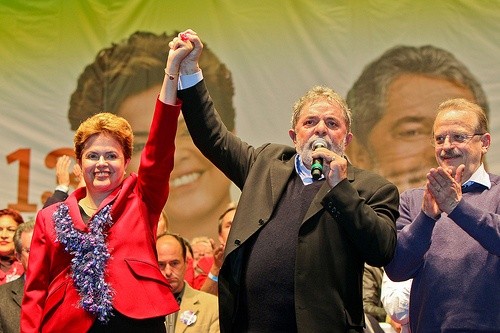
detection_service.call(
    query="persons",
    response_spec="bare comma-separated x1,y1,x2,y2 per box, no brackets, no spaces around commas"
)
384,98,500,333
173,29,399,333
19,32,194,333
67,31,235,248
343,44,490,193
0,156,411,333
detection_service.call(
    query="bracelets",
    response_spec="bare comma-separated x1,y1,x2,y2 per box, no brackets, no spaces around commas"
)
163,69,179,81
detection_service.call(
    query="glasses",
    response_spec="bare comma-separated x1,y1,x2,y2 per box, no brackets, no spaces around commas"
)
429,131,484,148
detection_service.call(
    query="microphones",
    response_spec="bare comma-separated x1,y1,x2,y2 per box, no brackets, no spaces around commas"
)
310,140,328,179
